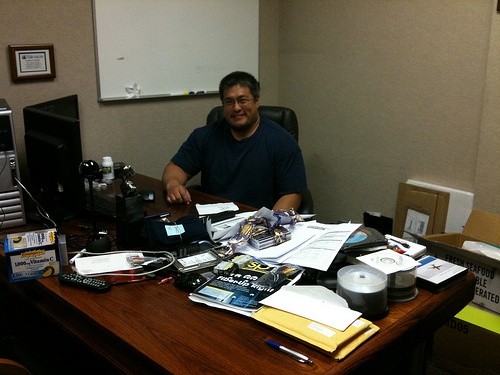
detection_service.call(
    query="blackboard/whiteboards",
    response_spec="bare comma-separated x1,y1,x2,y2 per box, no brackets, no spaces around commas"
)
91,0,261,103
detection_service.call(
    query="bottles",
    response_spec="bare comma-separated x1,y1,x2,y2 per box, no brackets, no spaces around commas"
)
102,156,115,180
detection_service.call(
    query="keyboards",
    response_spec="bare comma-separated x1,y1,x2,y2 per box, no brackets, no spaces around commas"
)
74,192,118,218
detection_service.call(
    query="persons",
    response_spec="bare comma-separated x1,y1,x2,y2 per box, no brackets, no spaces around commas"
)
163,71,306,214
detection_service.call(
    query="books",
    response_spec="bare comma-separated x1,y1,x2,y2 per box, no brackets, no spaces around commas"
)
192,254,305,312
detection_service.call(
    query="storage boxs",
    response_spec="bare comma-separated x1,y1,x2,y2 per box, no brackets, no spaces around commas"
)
247,226,290,250
400,209,500,375
4,228,62,284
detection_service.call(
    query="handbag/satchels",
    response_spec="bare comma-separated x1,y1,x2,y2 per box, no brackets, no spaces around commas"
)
129,213,208,249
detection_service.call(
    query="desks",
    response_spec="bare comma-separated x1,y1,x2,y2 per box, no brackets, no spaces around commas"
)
0,171,475,375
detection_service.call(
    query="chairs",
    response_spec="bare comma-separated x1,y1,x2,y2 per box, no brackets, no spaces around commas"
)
188,106,314,222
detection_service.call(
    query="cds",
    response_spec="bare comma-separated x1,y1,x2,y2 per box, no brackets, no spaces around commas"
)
343,229,368,244
338,272,415,316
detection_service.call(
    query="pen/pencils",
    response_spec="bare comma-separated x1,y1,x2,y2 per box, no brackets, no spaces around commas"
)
265,339,314,364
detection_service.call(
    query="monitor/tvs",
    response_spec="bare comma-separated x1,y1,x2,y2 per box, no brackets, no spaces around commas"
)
22,94,84,222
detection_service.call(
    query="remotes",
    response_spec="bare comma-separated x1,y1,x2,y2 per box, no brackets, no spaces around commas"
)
58,272,111,292
